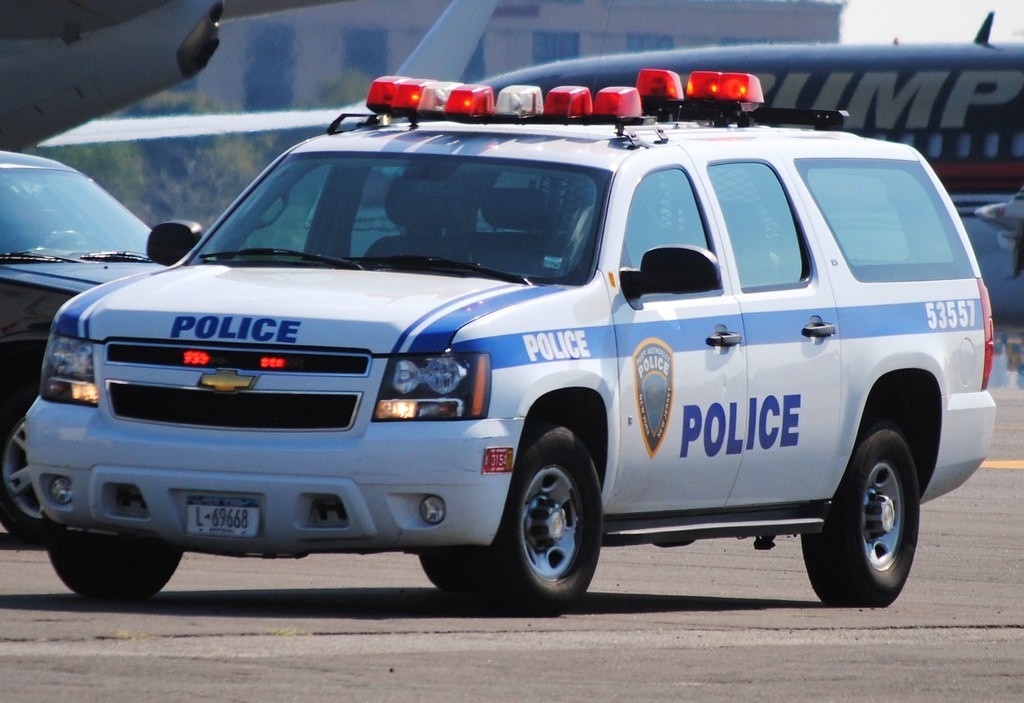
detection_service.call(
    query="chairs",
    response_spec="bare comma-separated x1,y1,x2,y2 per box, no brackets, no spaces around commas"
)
468,188,552,272
364,176,467,263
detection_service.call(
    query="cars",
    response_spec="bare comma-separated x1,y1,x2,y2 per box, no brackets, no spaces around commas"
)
1,151,193,542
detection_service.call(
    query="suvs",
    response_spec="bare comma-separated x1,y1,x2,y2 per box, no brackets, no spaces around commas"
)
1,64,998,619
